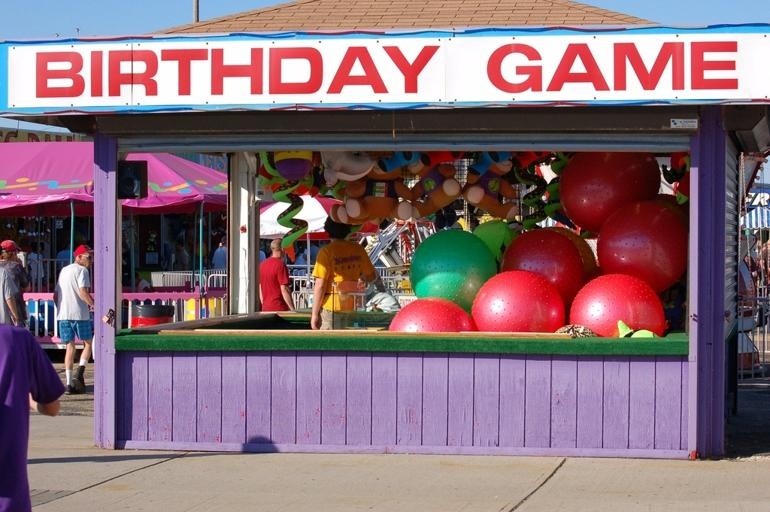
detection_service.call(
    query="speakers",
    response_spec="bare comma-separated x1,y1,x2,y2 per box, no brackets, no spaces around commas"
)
116,159,147,199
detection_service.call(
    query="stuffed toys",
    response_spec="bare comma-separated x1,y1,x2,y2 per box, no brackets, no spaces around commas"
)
274,149,574,228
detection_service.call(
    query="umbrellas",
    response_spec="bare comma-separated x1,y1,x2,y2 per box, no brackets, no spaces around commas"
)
259,191,380,291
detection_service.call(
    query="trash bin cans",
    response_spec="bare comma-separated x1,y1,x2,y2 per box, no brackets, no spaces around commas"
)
131,304,174,328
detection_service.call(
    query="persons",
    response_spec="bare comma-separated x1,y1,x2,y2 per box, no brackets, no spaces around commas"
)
1,235,28,326
50,246,94,397
0,309,66,512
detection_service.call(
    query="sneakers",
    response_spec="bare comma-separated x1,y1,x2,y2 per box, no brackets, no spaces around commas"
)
62,384,72,394
70,373,86,394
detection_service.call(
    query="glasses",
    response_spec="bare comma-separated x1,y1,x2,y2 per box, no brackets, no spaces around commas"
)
84,255,94,259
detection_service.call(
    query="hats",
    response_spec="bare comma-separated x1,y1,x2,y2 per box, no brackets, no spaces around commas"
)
0,240,20,250
72,243,96,253
